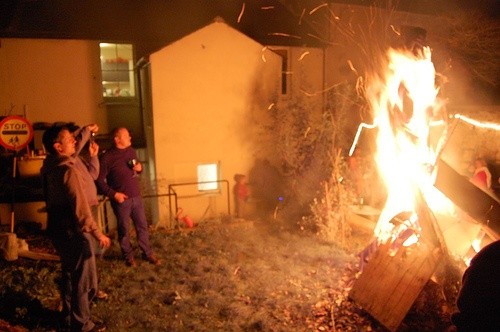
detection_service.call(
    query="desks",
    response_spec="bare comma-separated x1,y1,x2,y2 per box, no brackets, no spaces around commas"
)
0,175,109,232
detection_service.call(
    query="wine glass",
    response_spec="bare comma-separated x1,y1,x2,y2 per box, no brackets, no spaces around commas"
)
128,158,139,177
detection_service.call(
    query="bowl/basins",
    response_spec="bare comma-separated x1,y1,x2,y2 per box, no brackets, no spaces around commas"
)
16,155,46,176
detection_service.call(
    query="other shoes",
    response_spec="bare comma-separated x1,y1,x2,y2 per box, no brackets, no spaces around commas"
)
88,325,105,332
127,258,136,266
95,290,107,301
147,255,160,264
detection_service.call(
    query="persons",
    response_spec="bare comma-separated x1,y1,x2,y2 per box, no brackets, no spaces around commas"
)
96,126,159,267
40,124,111,332
65,122,100,227
449,141,500,332
